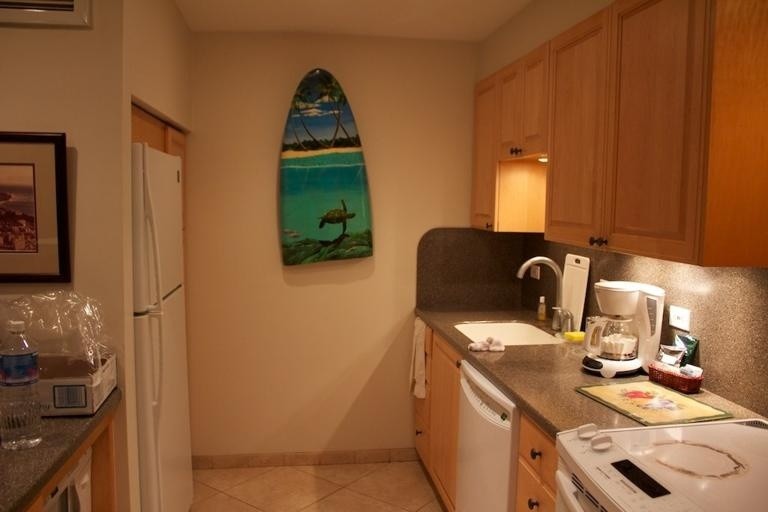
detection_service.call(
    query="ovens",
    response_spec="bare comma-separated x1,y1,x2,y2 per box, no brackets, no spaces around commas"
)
555,437,624,512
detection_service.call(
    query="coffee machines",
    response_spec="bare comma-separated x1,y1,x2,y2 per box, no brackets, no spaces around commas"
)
579,278,667,379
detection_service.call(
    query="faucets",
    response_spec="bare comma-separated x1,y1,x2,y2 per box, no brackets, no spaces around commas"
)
518,256,564,330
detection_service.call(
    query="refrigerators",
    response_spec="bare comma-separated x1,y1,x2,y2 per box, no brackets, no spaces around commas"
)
126,140,197,512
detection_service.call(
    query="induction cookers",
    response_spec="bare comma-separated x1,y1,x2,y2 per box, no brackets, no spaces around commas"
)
556,417,767,512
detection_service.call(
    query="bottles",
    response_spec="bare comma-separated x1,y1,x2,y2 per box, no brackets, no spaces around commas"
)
537,296,546,321
0,318,43,450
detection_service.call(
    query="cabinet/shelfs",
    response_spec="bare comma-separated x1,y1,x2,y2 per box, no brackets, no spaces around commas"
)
516,416,560,512
544,0,768,268
412,320,464,512
499,41,550,161
470,71,546,233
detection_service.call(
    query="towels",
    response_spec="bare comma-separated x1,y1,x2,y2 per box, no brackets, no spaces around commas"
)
409,316,426,400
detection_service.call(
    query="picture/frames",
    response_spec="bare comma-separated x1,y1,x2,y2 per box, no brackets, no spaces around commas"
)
1,2,93,28
0,132,71,285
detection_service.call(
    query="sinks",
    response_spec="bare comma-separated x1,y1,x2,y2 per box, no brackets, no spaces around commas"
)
455,317,563,346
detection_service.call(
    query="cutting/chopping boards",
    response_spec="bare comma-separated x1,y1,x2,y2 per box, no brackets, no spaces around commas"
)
551,252,591,334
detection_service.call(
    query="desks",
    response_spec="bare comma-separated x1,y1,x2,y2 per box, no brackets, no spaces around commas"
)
0,384,123,512
